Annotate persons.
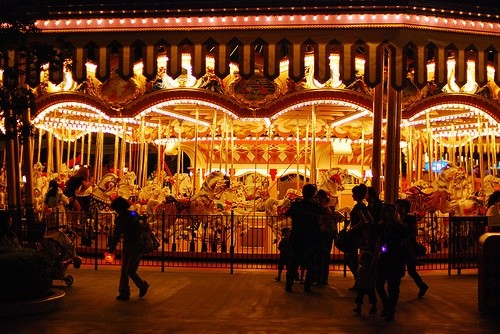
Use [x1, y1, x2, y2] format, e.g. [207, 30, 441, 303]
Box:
[0, 215, 23, 249]
[44, 167, 97, 246]
[105, 196, 160, 300]
[274, 184, 429, 321]
[485, 191, 500, 215]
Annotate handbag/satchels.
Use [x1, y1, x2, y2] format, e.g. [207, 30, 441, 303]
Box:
[335, 229, 360, 252]
[416, 241, 426, 258]
[137, 226, 160, 253]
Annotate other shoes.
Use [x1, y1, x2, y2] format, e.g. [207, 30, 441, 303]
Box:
[115, 294, 129, 301]
[284, 284, 291, 291]
[349, 286, 359, 291]
[379, 302, 395, 321]
[138, 280, 150, 298]
[275, 278, 280, 282]
[369, 306, 377, 315]
[352, 306, 361, 314]
[304, 286, 312, 295]
[418, 283, 429, 298]
[295, 273, 299, 282]
[299, 278, 304, 281]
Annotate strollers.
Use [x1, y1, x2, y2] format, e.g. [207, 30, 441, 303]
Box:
[26, 225, 75, 287]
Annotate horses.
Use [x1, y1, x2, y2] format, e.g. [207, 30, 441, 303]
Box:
[0, 162, 500, 256]
